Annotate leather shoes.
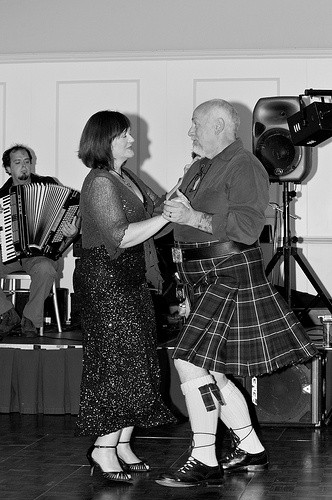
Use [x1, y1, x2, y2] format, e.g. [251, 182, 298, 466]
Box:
[162, 456, 225, 488]
[218, 445, 268, 472]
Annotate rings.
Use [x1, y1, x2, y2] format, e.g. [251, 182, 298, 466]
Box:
[169, 212, 172, 218]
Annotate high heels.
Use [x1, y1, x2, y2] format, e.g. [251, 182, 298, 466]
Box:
[114, 441, 151, 471]
[86, 442, 132, 481]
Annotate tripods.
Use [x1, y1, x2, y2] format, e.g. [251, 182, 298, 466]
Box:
[263, 184, 332, 313]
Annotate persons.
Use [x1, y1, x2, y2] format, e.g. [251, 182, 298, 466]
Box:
[0, 145, 80, 338]
[155, 99, 319, 487]
[73, 110, 190, 481]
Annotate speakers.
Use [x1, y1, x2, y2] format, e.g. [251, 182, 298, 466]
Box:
[246, 360, 313, 427]
[251, 97, 312, 184]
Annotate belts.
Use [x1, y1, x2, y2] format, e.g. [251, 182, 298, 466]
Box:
[171, 238, 264, 262]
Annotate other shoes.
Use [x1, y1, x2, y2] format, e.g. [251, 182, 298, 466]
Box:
[63, 320, 81, 332]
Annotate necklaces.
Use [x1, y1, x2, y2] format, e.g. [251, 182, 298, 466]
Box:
[110, 168, 131, 188]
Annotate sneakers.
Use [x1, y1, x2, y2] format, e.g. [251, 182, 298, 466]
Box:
[20, 315, 37, 338]
[0, 307, 21, 336]
